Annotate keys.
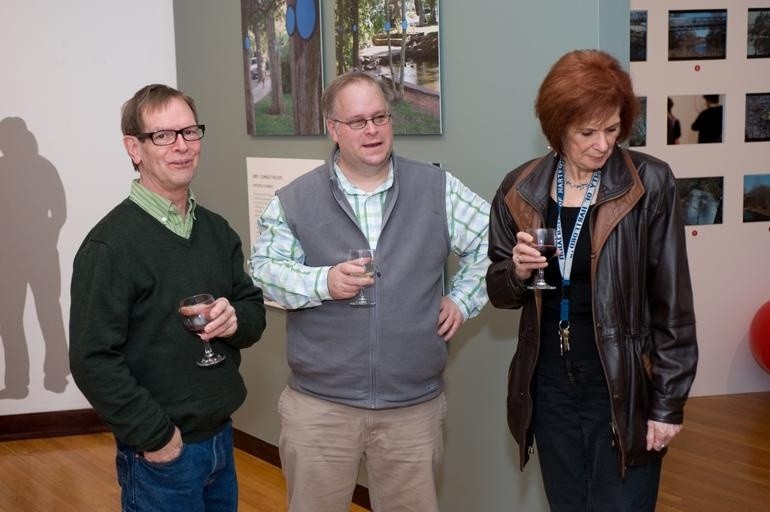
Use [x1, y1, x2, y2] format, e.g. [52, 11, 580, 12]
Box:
[557, 319, 571, 357]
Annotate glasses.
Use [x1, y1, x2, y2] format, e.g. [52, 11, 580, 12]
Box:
[333, 113, 391, 129]
[137, 125, 205, 146]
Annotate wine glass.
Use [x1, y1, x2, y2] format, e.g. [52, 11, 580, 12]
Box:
[522, 226, 562, 289]
[347, 251, 380, 308]
[179, 296, 228, 367]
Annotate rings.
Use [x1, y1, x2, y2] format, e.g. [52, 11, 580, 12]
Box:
[656, 440, 665, 448]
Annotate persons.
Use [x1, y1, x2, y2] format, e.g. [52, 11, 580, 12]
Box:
[69, 86, 267, 512]
[485, 49, 698, 512]
[248, 73, 493, 512]
[665, 94, 723, 145]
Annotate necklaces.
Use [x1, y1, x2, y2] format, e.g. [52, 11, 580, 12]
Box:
[562, 158, 592, 190]
